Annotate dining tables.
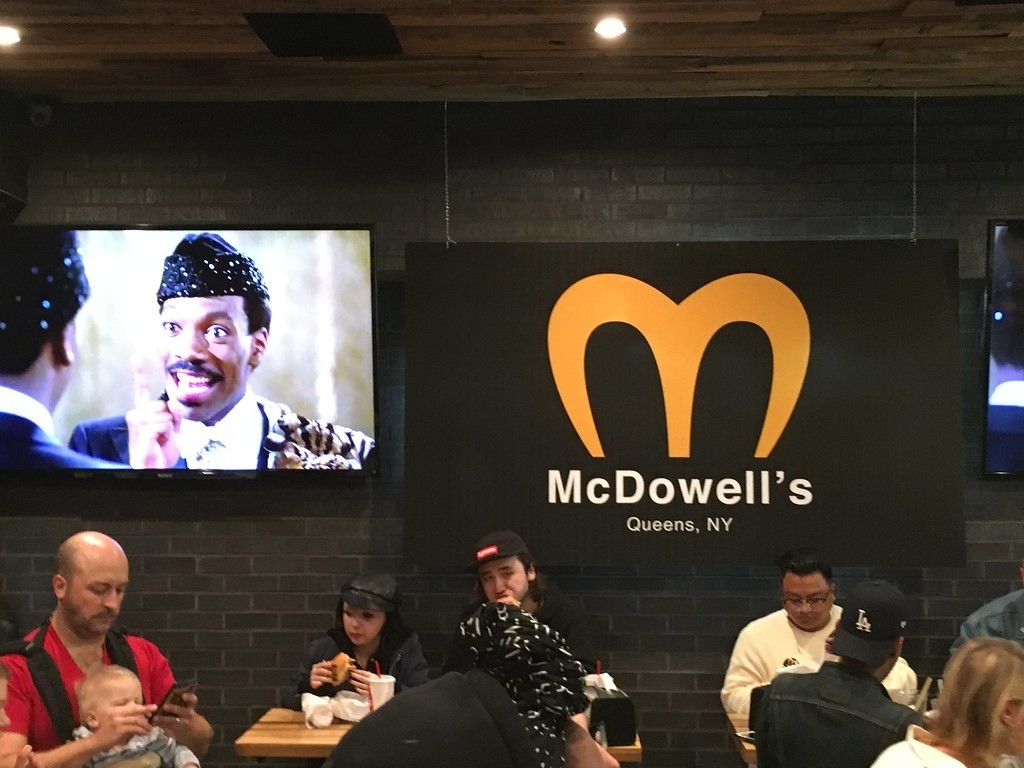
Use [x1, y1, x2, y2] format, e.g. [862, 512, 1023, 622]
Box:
[235, 707, 643, 768]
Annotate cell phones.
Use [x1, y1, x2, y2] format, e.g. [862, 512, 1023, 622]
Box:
[149, 680, 198, 725]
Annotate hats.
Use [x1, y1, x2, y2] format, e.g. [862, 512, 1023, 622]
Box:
[340, 570, 403, 613]
[463, 530, 528, 571]
[157, 231, 268, 305]
[322, 670, 539, 768]
[830, 580, 909, 668]
[0, 231, 90, 349]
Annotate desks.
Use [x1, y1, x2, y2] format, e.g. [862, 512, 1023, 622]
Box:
[726, 710, 758, 764]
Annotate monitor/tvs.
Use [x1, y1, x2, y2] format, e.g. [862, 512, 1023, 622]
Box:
[983, 216, 1024, 480]
[0, 223, 380, 480]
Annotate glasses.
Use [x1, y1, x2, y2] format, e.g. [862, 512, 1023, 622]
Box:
[781, 583, 831, 612]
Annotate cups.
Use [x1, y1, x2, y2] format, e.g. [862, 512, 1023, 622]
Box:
[368, 674, 397, 713]
[897, 686, 940, 716]
[584, 673, 617, 742]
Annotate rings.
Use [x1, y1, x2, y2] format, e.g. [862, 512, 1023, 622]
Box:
[176, 718, 180, 722]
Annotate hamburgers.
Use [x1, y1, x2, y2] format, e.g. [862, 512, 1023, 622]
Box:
[331, 652, 355, 684]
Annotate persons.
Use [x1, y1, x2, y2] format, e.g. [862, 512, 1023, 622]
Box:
[0, 531, 213, 768]
[0, 663, 39, 767]
[720, 548, 918, 712]
[755, 580, 925, 768]
[0, 231, 127, 470]
[65, 665, 200, 768]
[323, 602, 619, 768]
[436, 531, 602, 676]
[949, 556, 1024, 652]
[869, 637, 1024, 768]
[291, 572, 433, 710]
[68, 234, 374, 470]
